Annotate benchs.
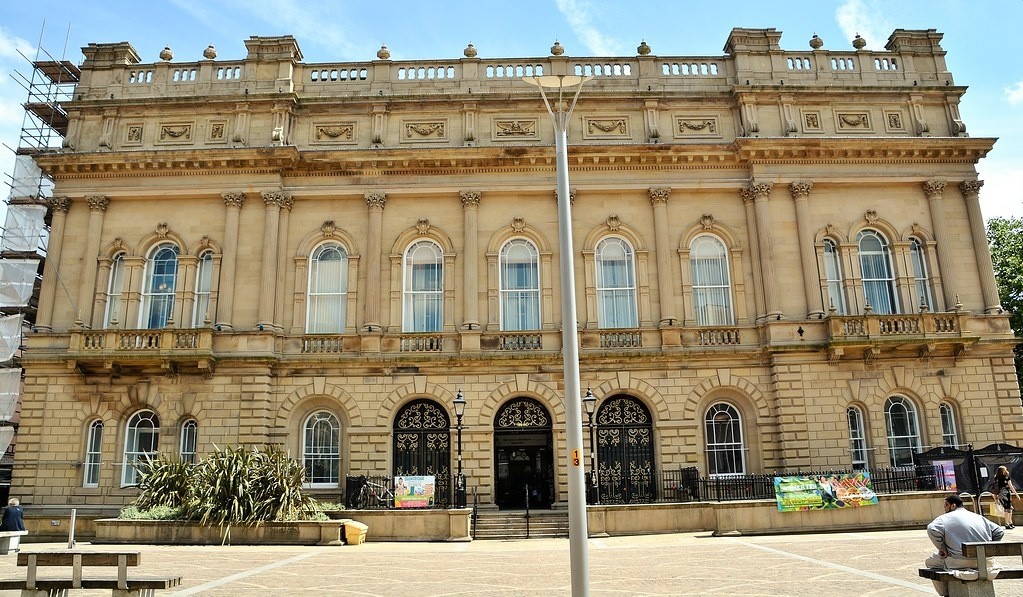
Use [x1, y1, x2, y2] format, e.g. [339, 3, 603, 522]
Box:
[0, 530, 28, 555]
[919, 542, 1023, 597]
[0, 551, 183, 597]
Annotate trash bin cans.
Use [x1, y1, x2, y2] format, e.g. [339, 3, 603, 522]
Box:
[344, 521, 369, 545]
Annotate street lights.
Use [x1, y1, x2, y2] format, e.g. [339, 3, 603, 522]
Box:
[452, 388, 467, 509]
[582, 386, 599, 505]
[522, 75, 595, 597]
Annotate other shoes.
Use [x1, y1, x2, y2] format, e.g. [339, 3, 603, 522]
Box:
[1005, 523, 1015, 529]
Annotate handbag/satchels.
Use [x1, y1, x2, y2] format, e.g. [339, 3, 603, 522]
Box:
[991, 481, 999, 495]
[989, 502, 1005, 518]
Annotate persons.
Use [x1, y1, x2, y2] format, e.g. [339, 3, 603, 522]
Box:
[992, 465, 1021, 529]
[0, 498, 25, 552]
[925, 494, 1005, 597]
[818, 476, 836, 503]
[395, 477, 407, 496]
